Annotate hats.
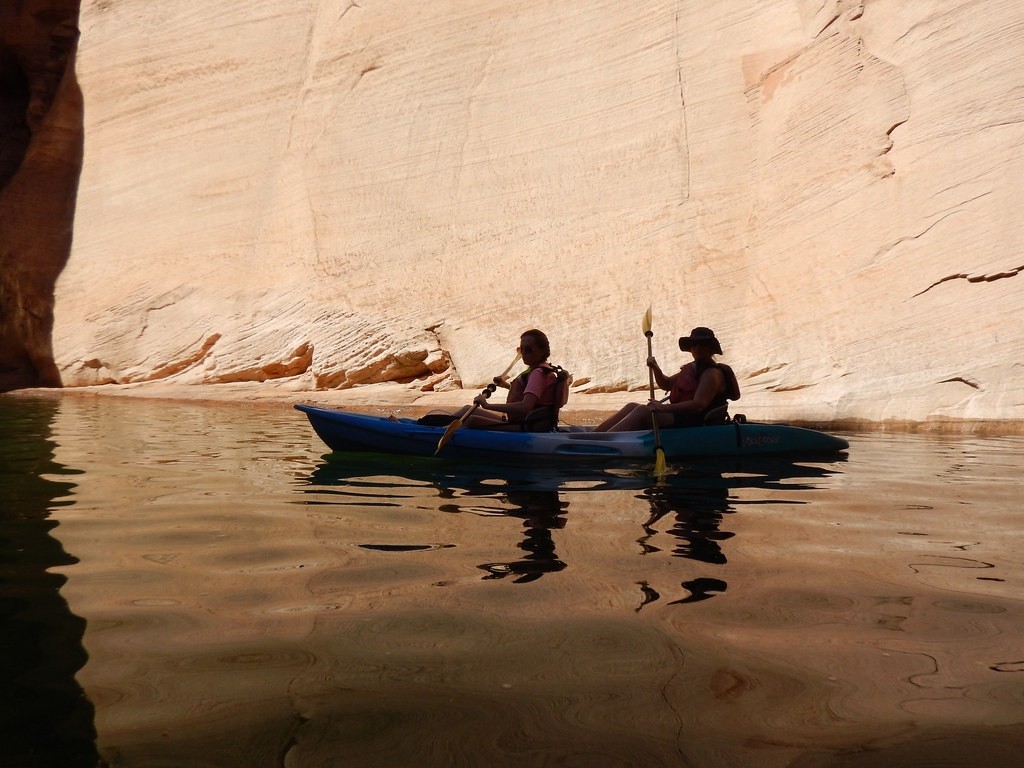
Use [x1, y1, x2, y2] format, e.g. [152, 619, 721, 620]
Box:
[679, 327, 722, 355]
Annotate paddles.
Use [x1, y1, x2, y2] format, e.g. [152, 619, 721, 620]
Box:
[641, 301, 666, 479]
[434, 350, 525, 455]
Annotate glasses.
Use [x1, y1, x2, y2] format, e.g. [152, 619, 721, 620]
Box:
[691, 342, 709, 348]
[517, 346, 542, 354]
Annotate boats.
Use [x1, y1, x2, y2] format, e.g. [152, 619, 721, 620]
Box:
[293, 404, 852, 468]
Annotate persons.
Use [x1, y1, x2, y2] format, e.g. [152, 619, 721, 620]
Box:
[388, 329, 571, 424]
[597, 327, 741, 431]
